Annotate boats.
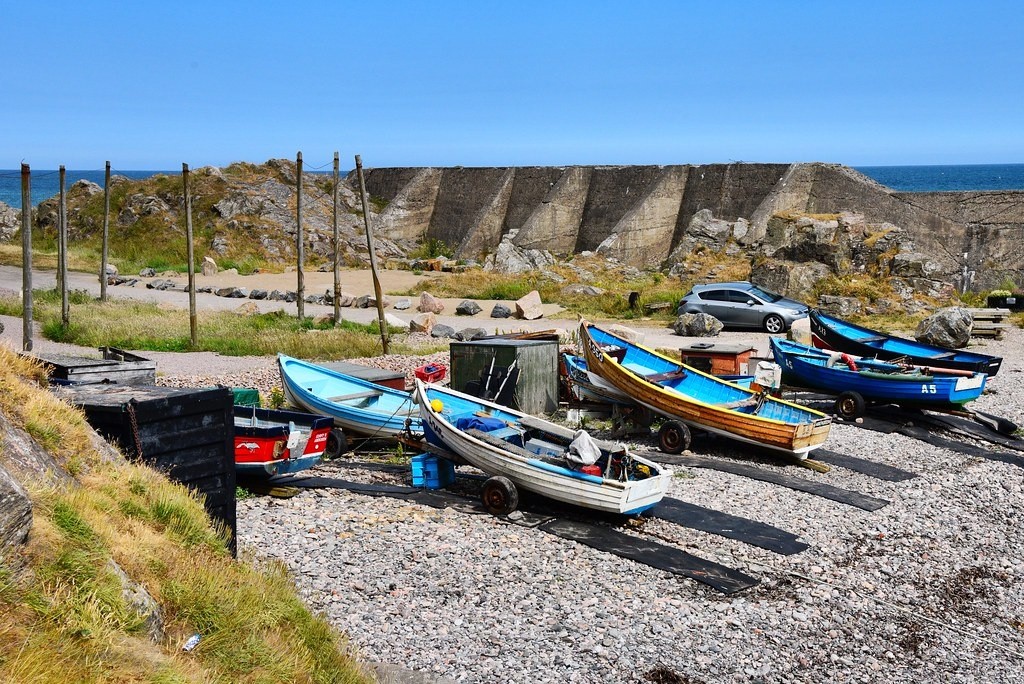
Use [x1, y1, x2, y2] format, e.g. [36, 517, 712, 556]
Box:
[275, 350, 428, 440]
[806, 305, 1004, 381]
[561, 352, 757, 407]
[578, 316, 833, 455]
[413, 376, 672, 517]
[232, 402, 334, 476]
[768, 333, 990, 406]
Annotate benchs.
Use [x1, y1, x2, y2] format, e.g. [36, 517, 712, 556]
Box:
[973, 316, 1008, 323]
[969, 324, 1011, 340]
[327, 390, 384, 403]
[714, 397, 760, 410]
[488, 424, 532, 438]
[932, 351, 956, 360]
[600, 344, 627, 352]
[855, 335, 887, 342]
[647, 370, 688, 383]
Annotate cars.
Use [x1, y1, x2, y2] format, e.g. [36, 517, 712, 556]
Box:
[678, 281, 811, 334]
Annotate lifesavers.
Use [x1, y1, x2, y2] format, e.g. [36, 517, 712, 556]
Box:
[826, 352, 858, 371]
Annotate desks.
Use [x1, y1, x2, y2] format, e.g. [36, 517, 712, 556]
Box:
[962, 308, 1011, 323]
[206, 386, 263, 408]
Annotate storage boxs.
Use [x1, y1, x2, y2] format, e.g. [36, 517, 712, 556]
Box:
[415, 362, 446, 383]
